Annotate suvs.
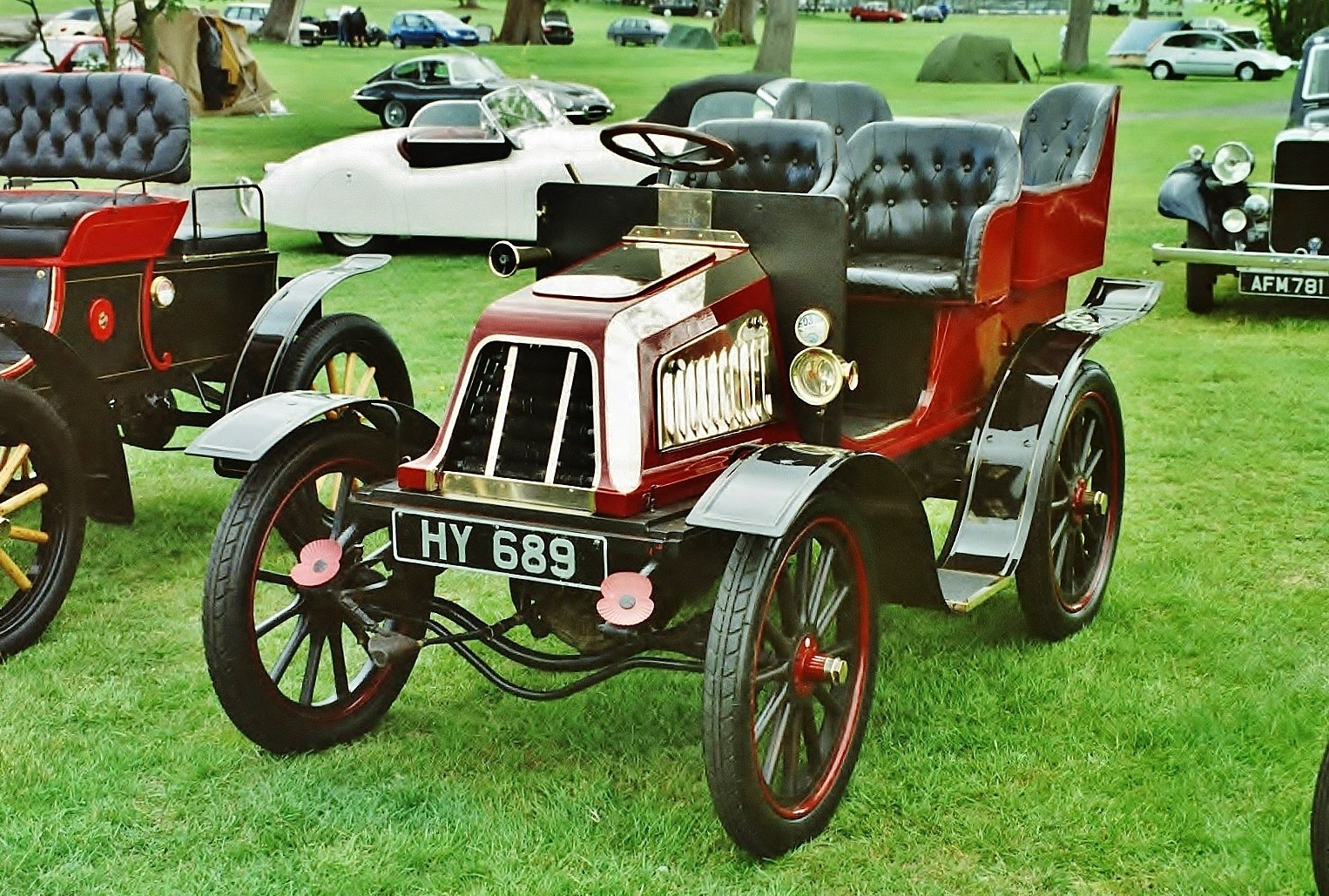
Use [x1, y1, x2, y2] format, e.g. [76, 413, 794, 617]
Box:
[222, 1, 321, 47]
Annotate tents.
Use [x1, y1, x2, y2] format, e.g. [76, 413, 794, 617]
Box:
[88, 0, 280, 122]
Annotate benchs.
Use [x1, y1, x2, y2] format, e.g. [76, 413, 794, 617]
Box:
[0, 71, 192, 258]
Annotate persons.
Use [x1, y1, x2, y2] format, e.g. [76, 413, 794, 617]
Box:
[338, 11, 350, 47]
[198, 17, 224, 111]
[350, 6, 367, 48]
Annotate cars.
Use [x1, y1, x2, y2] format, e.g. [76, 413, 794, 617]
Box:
[850, 2, 908, 23]
[912, 5, 944, 23]
[181, 80, 1165, 859]
[0, 7, 179, 98]
[387, 9, 480, 50]
[540, 9, 574, 45]
[0, 71, 417, 664]
[607, 16, 670, 47]
[299, 5, 384, 47]
[621, 0, 726, 18]
[1150, 23, 1329, 317]
[1143, 30, 1294, 82]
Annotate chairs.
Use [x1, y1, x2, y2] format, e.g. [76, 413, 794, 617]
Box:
[670, 78, 1123, 302]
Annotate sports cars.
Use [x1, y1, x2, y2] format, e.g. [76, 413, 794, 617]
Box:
[350, 54, 614, 136]
